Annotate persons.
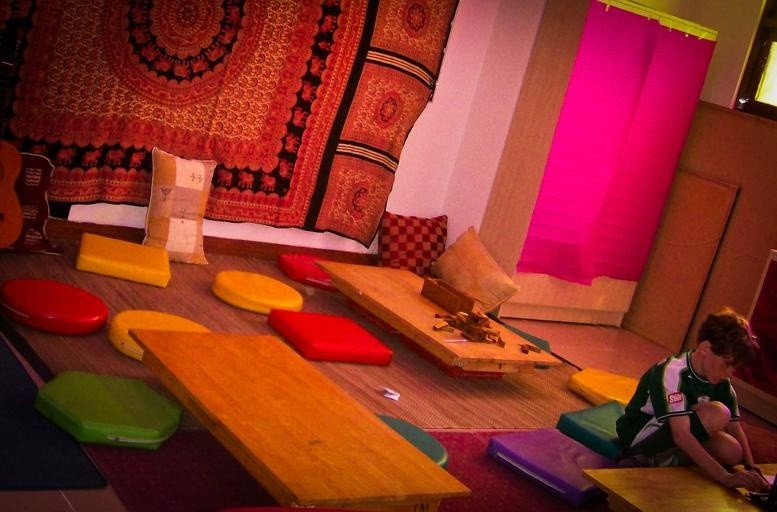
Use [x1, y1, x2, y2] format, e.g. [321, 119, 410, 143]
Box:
[616, 304, 775, 501]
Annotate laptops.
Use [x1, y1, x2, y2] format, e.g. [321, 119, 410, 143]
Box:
[748, 473, 777, 512]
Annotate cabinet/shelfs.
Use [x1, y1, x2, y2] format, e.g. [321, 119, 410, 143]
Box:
[473, 0, 720, 328]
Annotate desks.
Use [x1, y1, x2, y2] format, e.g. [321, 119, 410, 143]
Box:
[583, 461, 776, 512]
[317, 258, 561, 387]
[127, 318, 471, 509]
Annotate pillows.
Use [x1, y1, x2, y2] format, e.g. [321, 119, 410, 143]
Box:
[378, 209, 521, 317]
[141, 147, 217, 265]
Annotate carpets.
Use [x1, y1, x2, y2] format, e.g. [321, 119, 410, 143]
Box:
[91, 429, 608, 511]
[0, 329, 108, 491]
